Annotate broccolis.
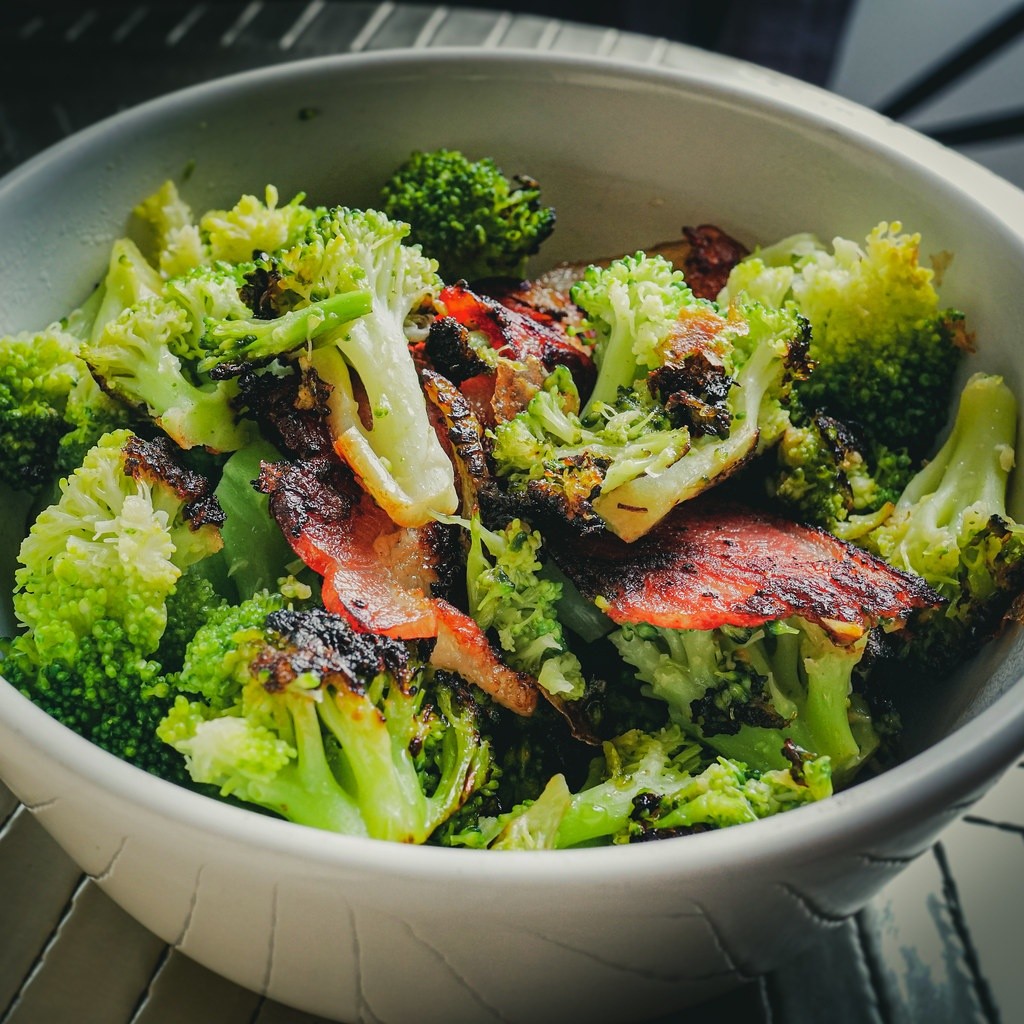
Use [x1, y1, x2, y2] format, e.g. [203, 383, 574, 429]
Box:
[1, 147, 1024, 845]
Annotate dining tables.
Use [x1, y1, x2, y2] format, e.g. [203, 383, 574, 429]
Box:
[0, 4, 1024, 1023]
